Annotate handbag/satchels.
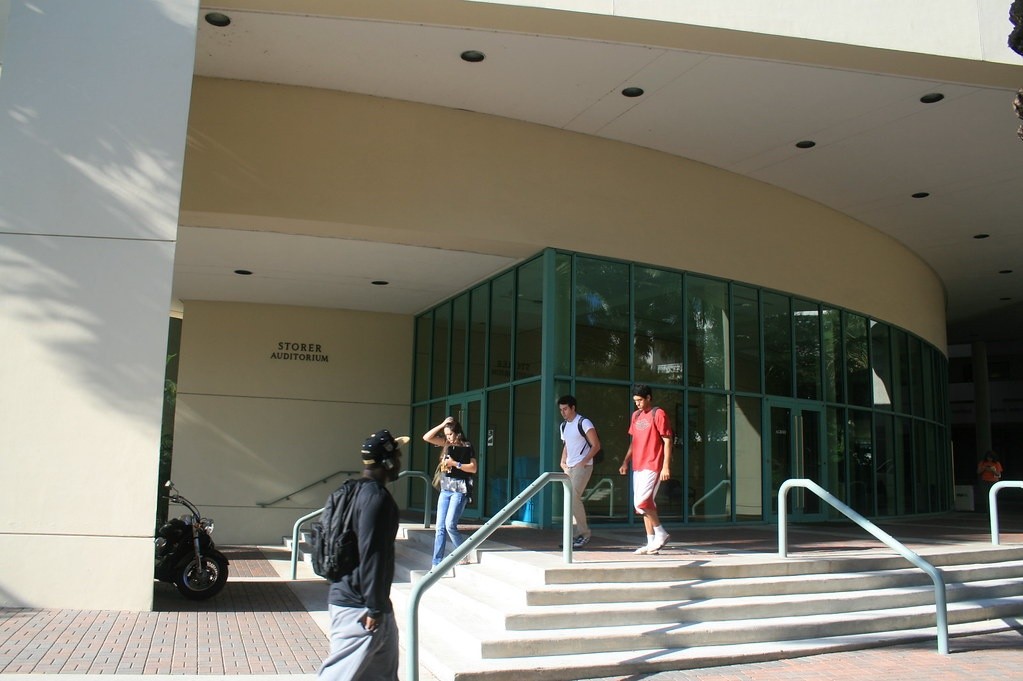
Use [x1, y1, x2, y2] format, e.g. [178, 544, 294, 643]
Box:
[432, 469, 441, 491]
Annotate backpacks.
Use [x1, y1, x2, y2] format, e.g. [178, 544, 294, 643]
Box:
[311, 477, 370, 582]
[562, 417, 604, 464]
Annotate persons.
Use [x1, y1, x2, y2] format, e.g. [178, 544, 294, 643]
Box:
[318, 430, 410, 681]
[619, 385, 672, 555]
[423, 417, 477, 576]
[977, 452, 1003, 517]
[558, 394, 601, 548]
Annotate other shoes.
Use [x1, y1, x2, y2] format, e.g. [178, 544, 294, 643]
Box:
[456, 556, 468, 564]
[421, 570, 433, 578]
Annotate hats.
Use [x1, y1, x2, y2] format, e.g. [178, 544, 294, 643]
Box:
[361, 429, 411, 464]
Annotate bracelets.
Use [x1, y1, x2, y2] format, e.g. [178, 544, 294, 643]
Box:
[457, 462, 461, 469]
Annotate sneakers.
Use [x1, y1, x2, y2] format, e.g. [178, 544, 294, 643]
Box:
[573, 535, 592, 548]
[559, 537, 578, 549]
[634, 544, 658, 555]
[646, 531, 670, 552]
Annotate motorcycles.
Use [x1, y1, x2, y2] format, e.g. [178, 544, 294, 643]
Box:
[155, 480, 230, 602]
